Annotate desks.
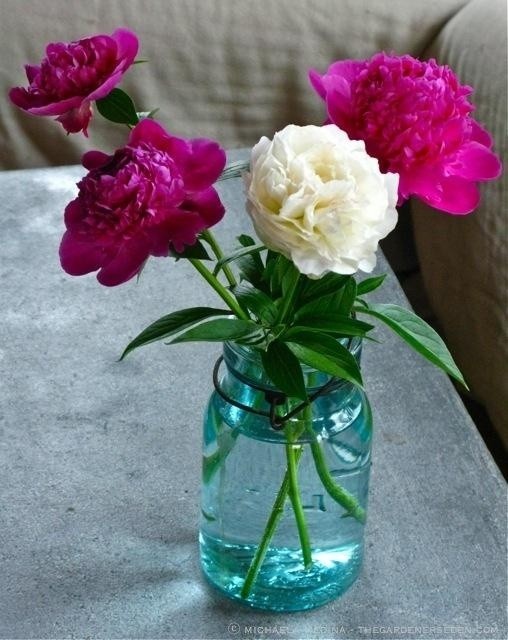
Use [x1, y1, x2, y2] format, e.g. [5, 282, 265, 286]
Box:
[1, 145, 508, 638]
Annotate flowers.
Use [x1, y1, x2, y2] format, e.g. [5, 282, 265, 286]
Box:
[9, 25, 504, 596]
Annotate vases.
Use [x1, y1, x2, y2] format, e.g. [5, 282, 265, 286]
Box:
[194, 332, 373, 613]
